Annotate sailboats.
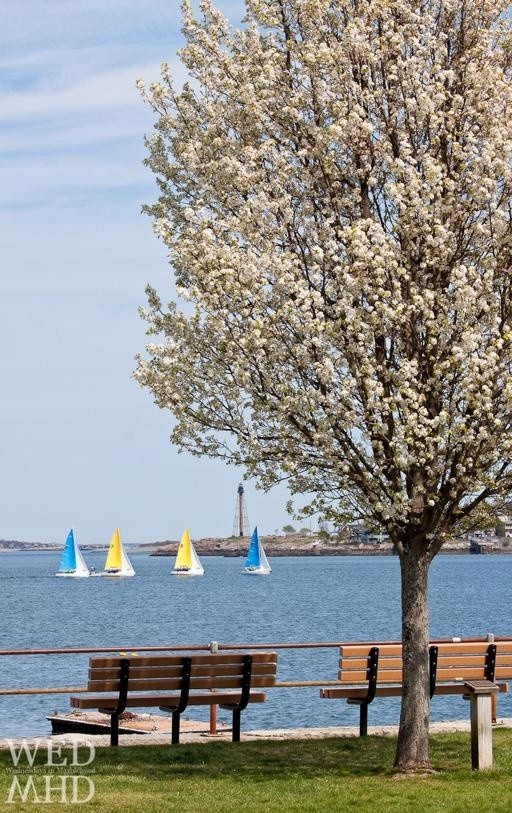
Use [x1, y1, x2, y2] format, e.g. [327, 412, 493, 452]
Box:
[93, 525, 136, 576]
[54, 529, 90, 578]
[171, 528, 204, 576]
[241, 526, 273, 575]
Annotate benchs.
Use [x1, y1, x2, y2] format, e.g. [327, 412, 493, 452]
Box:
[319, 640, 512, 738]
[68, 650, 279, 748]
